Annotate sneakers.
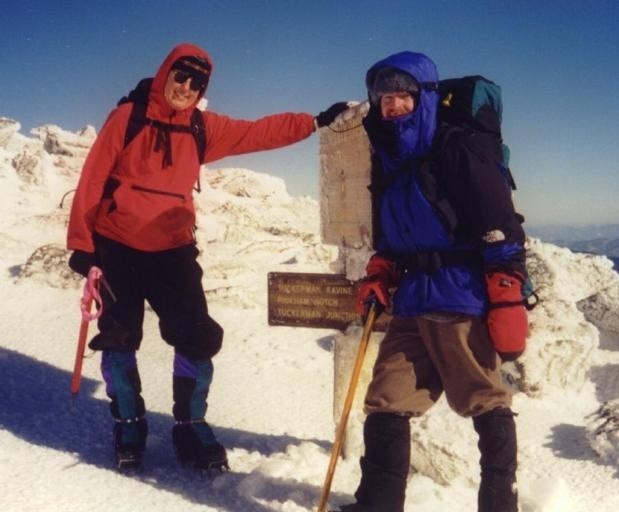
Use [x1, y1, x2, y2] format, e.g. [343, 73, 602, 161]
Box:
[177, 454, 227, 473]
[116, 439, 142, 470]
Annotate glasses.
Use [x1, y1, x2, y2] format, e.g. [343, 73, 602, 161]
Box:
[173, 69, 202, 91]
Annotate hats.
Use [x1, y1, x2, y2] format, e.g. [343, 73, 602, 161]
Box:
[171, 55, 210, 84]
[374, 68, 418, 96]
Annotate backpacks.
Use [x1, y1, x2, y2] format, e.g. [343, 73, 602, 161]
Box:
[423, 75, 525, 224]
[117, 77, 205, 167]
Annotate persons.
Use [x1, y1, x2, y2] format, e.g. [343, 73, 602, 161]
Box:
[66, 43, 361, 468]
[339, 49, 541, 512]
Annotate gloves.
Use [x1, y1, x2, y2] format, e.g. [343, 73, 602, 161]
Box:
[486, 268, 528, 360]
[356, 256, 396, 325]
[69, 249, 99, 277]
[317, 101, 349, 127]
